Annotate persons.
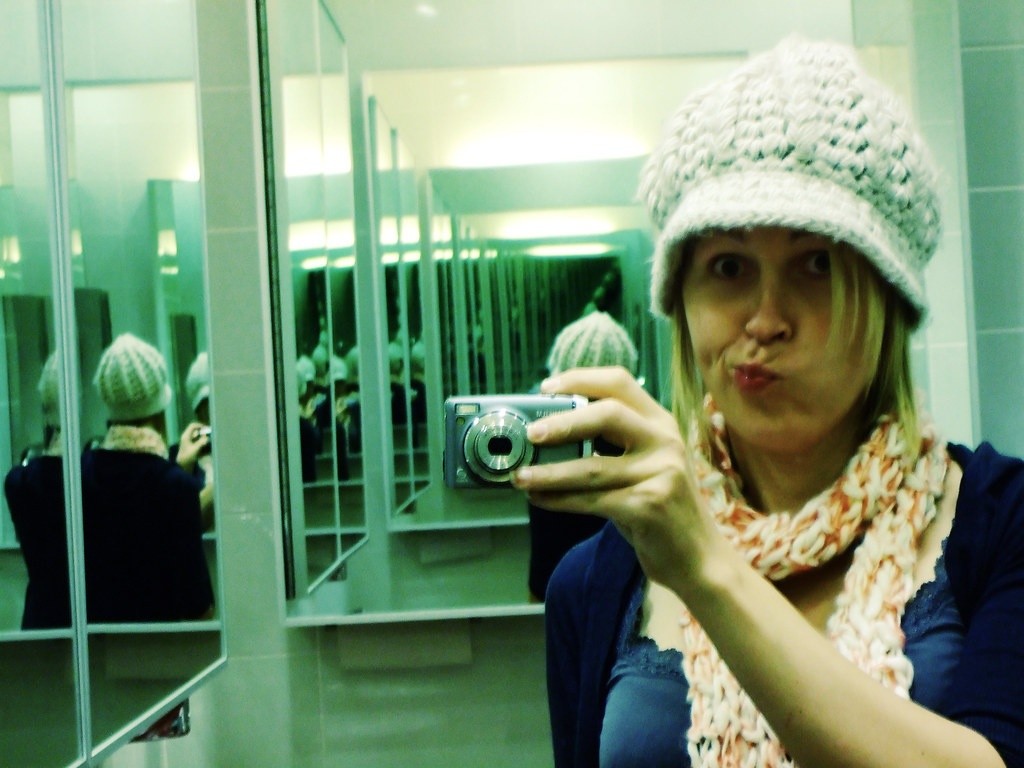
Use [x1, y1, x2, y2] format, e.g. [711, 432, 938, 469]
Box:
[524, 310, 641, 603]
[4, 330, 426, 639]
[508, 34, 1024, 768]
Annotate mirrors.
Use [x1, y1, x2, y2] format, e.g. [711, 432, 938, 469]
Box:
[0, 1, 229, 768]
[1, 0, 650, 643]
[254, 1, 979, 630]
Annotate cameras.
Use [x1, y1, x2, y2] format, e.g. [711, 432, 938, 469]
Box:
[342, 393, 359, 406]
[312, 394, 327, 409]
[191, 427, 213, 454]
[442, 393, 591, 490]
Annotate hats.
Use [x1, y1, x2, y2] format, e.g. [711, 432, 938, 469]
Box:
[546, 312, 638, 381]
[298, 334, 428, 381]
[638, 36, 941, 327]
[39, 350, 61, 424]
[95, 333, 172, 420]
[186, 352, 210, 410]
